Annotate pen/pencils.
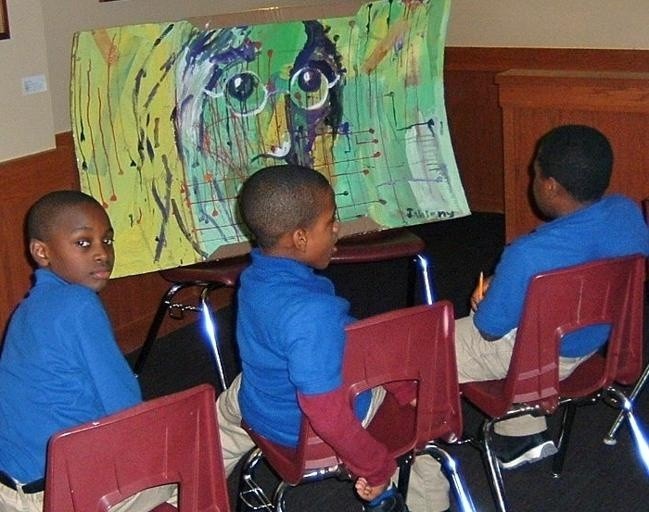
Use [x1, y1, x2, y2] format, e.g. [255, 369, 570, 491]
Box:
[478, 270, 484, 300]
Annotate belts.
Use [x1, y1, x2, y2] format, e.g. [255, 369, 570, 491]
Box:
[0, 470, 45, 494]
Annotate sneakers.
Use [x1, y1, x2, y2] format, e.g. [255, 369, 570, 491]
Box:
[362, 480, 407, 512]
[495, 428, 559, 471]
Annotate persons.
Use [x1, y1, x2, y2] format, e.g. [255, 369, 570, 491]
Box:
[454, 120, 649, 474]
[211, 161, 456, 511]
[0, 189, 146, 512]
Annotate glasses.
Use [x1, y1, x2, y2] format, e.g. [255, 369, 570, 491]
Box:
[202, 65, 341, 119]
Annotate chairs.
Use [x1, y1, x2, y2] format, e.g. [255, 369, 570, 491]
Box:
[239, 301, 478, 512]
[130, 260, 239, 394]
[465, 252, 649, 512]
[44, 385, 232, 511]
[327, 228, 435, 304]
